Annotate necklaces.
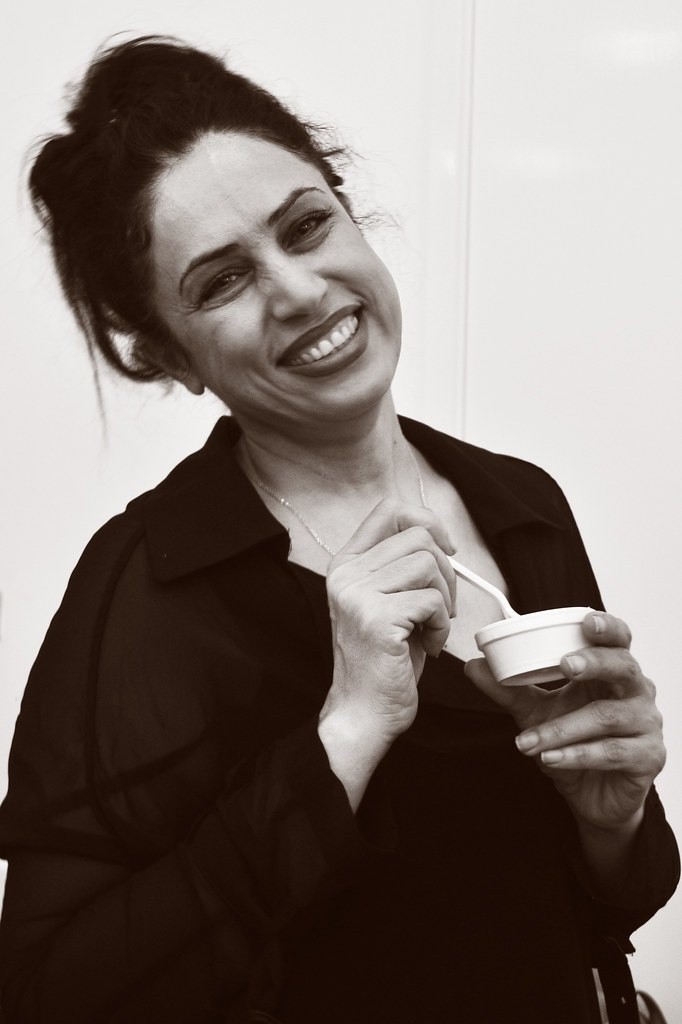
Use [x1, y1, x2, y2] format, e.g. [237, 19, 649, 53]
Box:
[243, 446, 427, 552]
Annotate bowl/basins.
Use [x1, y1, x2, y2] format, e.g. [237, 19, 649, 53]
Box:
[474, 607, 602, 686]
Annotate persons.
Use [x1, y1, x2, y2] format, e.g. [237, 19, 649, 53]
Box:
[0, 33, 681, 1024]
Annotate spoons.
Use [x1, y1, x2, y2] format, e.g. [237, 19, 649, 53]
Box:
[445, 553, 522, 619]
